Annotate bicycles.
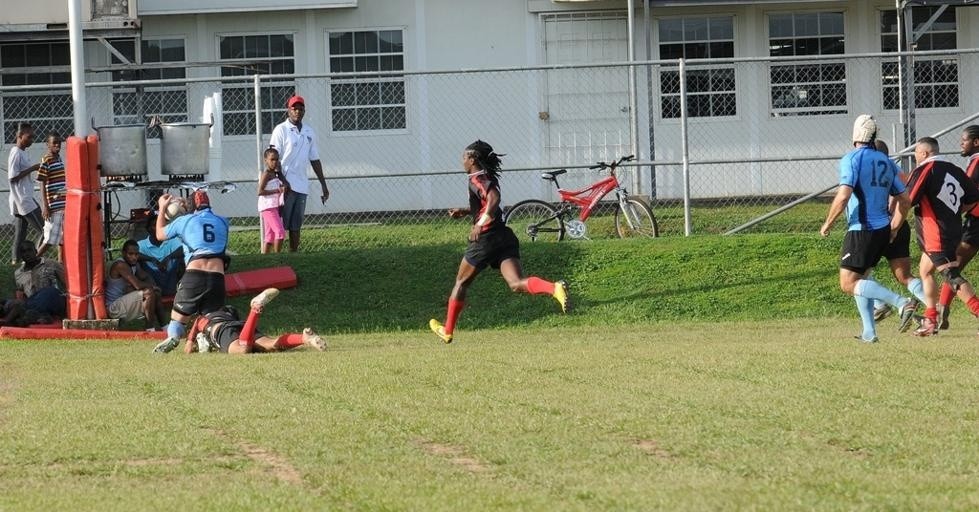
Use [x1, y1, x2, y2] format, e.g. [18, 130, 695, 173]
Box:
[506, 155, 658, 239]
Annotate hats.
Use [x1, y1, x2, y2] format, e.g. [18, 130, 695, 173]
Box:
[288, 96, 304, 107]
[187, 190, 209, 209]
[852, 114, 877, 145]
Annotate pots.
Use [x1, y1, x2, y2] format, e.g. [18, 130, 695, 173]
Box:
[90, 115, 156, 177]
[156, 112, 215, 176]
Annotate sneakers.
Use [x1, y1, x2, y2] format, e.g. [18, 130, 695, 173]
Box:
[153, 337, 178, 354]
[855, 301, 949, 342]
[429, 319, 453, 343]
[303, 328, 326, 350]
[196, 334, 212, 353]
[554, 281, 571, 314]
[250, 288, 279, 307]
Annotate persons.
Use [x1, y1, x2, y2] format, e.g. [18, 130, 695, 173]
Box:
[0, 239, 65, 326]
[819, 114, 920, 344]
[912, 125, 979, 330]
[903, 137, 979, 339]
[104, 189, 327, 355]
[35, 132, 67, 266]
[866, 139, 950, 329]
[429, 140, 571, 344]
[256, 145, 291, 255]
[7, 122, 45, 266]
[269, 96, 329, 254]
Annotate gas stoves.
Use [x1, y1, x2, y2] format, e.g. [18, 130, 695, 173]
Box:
[166, 174, 206, 184]
[104, 174, 143, 184]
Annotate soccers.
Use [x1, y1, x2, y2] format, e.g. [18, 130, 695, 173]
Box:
[195, 332, 211, 352]
[165, 199, 188, 219]
